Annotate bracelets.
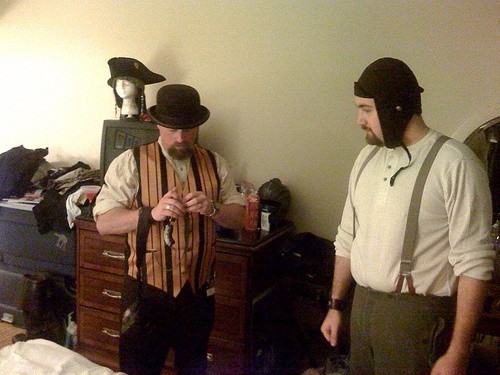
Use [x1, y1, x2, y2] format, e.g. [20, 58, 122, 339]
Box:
[327, 299, 344, 309]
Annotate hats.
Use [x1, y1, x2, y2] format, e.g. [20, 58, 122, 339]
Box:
[106, 56, 167, 85]
[354, 57, 423, 149]
[147, 84, 211, 129]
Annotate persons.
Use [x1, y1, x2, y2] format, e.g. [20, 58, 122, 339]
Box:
[93, 84, 247, 375]
[107, 57, 167, 115]
[320, 58, 496, 375]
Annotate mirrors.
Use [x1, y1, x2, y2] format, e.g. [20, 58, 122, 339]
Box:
[450, 108, 500, 225]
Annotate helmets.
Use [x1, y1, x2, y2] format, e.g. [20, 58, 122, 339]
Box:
[258, 178, 291, 230]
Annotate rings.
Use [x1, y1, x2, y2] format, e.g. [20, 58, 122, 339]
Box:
[167, 205, 170, 210]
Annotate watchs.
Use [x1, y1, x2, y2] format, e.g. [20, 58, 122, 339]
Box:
[208, 200, 219, 217]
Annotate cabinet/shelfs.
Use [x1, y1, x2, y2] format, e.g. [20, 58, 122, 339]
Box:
[76, 216, 296, 375]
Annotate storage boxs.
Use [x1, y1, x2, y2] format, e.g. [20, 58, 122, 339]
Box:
[0, 195, 77, 276]
